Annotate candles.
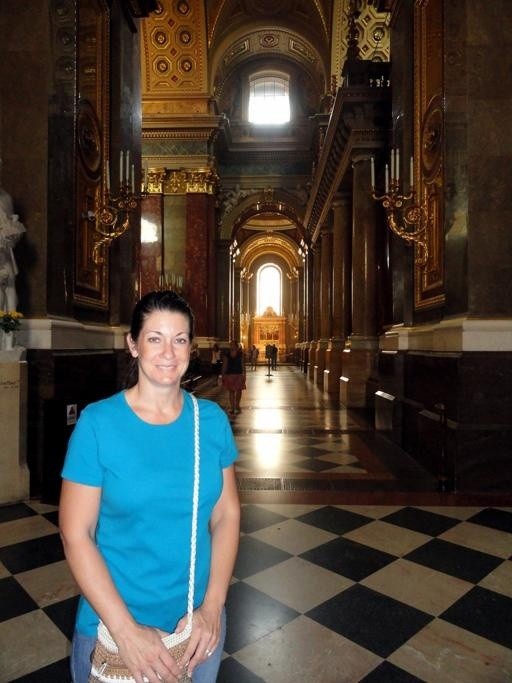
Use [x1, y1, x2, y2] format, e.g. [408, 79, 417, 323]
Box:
[370, 148, 415, 193]
[103, 149, 149, 195]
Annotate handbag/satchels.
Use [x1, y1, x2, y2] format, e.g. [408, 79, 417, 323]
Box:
[87, 616, 193, 683]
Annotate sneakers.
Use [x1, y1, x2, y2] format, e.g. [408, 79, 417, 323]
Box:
[227, 405, 241, 414]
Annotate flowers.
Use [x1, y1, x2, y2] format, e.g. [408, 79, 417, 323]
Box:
[1, 311, 22, 333]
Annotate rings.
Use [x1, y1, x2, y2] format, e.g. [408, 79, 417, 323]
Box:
[207, 651, 214, 657]
[205, 648, 210, 653]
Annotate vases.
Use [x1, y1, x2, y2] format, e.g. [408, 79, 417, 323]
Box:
[5, 334, 14, 350]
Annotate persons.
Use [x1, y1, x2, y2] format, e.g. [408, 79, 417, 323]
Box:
[249, 344, 259, 371]
[208, 343, 222, 386]
[221, 340, 247, 413]
[61, 290, 242, 683]
[270, 343, 278, 370]
